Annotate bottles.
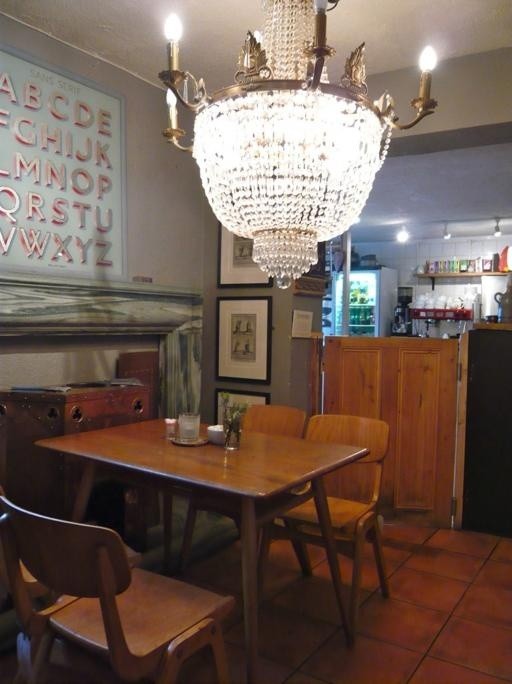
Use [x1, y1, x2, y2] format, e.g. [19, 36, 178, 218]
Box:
[349, 307, 370, 324]
[495, 286, 512, 323]
[350, 280, 369, 304]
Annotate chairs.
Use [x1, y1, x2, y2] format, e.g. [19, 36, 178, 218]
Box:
[177, 404, 389, 648]
[0, 495, 235, 683]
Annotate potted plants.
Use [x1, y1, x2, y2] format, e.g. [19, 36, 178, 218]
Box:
[218, 392, 249, 452]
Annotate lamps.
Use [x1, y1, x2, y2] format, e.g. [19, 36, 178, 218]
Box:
[159, 0, 438, 291]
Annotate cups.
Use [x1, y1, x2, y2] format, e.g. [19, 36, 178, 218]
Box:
[179, 412, 201, 442]
[414, 318, 473, 339]
[416, 292, 456, 310]
[485, 316, 498, 324]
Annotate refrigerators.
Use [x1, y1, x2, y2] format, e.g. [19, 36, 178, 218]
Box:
[334, 268, 398, 337]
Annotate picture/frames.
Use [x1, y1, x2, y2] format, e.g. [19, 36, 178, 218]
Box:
[0, 42, 128, 283]
[214, 222, 273, 431]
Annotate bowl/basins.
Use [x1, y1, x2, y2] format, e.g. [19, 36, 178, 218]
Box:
[207, 425, 241, 445]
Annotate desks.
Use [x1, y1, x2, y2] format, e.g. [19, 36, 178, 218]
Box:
[33, 418, 371, 683]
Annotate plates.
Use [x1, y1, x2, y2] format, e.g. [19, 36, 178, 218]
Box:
[171, 438, 209, 447]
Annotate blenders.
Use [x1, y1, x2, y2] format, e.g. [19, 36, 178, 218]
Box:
[391, 286, 413, 335]
